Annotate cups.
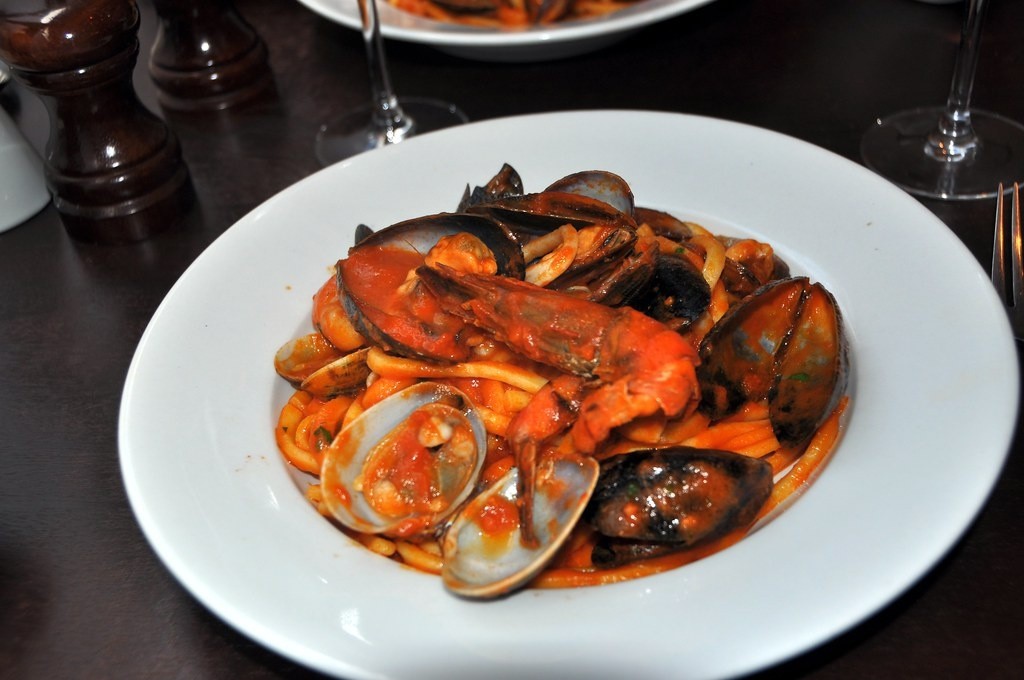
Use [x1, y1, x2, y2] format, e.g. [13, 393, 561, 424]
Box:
[1, 106, 50, 236]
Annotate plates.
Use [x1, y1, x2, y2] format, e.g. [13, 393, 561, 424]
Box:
[115, 111, 1023, 679]
[299, 0, 710, 45]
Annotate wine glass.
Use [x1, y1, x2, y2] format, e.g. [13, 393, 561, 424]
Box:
[859, 0, 1024, 199]
[313, 0, 470, 161]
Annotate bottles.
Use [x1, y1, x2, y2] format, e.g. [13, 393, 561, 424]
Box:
[147, 0, 270, 112]
[0, 0, 186, 226]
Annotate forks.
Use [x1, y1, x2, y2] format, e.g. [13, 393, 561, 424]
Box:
[992, 183, 1024, 380]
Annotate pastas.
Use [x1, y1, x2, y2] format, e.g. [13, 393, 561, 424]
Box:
[276, 225, 850, 585]
[386, 0, 636, 26]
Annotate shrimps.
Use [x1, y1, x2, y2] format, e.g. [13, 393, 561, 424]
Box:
[427, 261, 698, 456]
